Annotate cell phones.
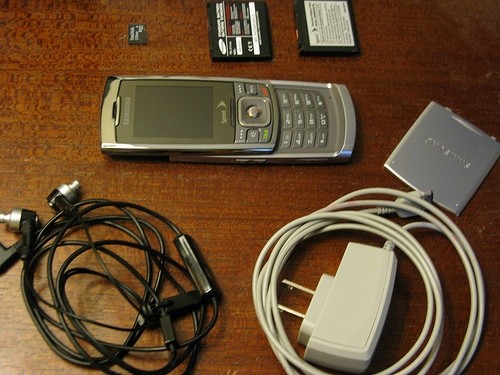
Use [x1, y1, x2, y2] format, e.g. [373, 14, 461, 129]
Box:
[100, 74, 358, 164]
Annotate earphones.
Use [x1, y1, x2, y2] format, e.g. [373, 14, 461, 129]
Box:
[45, 180, 81, 213]
[0, 209, 39, 257]
